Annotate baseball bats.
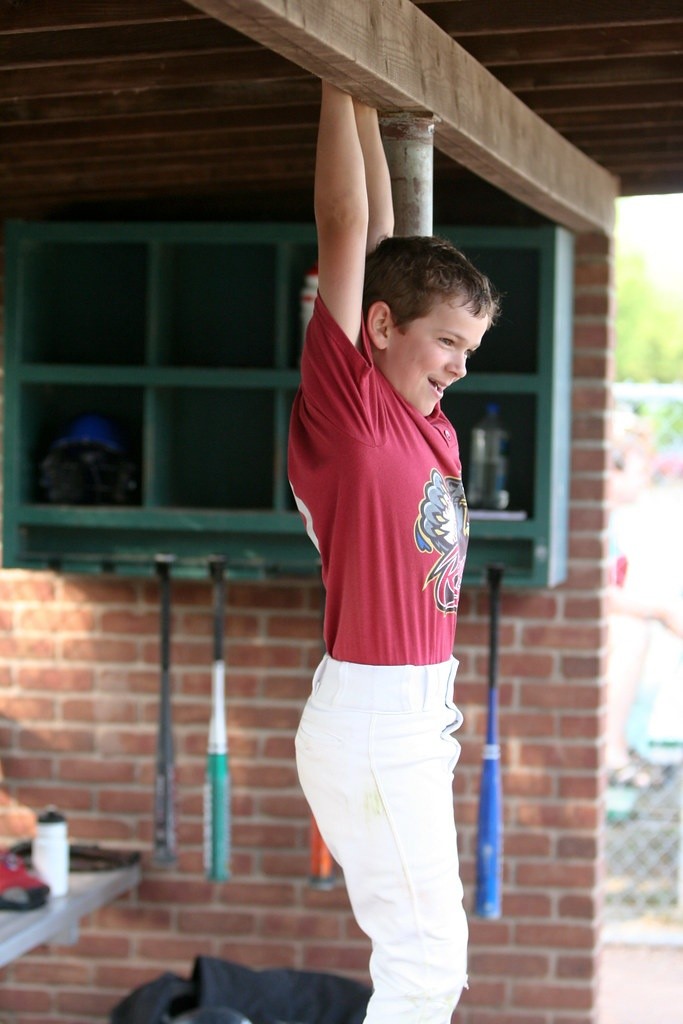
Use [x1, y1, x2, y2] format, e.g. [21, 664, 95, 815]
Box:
[304, 817, 337, 893]
[471, 562, 507, 920]
[142, 560, 177, 865]
[199, 558, 232, 880]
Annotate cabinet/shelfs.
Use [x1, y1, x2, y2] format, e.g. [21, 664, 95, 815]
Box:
[0, 221, 573, 594]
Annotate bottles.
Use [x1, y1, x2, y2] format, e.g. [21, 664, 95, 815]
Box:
[464, 406, 511, 511]
[28, 803, 70, 900]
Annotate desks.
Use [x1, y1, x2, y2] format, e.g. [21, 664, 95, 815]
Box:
[0, 865, 145, 972]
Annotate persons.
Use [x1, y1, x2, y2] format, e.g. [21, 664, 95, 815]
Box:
[287, 78, 496, 1024]
